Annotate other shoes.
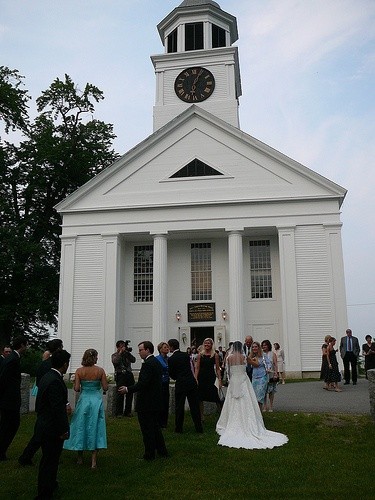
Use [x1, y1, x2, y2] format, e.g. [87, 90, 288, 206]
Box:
[113, 414, 122, 419]
[262, 407, 267, 412]
[282, 380, 285, 384]
[136, 457, 148, 462]
[268, 408, 272, 412]
[353, 382, 357, 385]
[124, 413, 134, 417]
[20, 460, 35, 466]
[344, 382, 350, 385]
[323, 384, 342, 392]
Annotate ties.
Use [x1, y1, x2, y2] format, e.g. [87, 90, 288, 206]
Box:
[348, 337, 351, 351]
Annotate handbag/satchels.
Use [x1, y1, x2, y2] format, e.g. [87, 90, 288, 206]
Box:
[266, 371, 280, 383]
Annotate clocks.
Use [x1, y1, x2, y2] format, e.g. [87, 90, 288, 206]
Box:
[173, 67, 215, 103]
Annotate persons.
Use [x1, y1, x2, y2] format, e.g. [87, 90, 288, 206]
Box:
[112, 340, 136, 418]
[215, 341, 289, 450]
[63, 348, 109, 471]
[362, 335, 375, 379]
[36, 349, 71, 500]
[195, 338, 222, 405]
[155, 342, 170, 414]
[319, 335, 342, 392]
[243, 336, 286, 413]
[339, 329, 360, 385]
[0, 336, 63, 465]
[187, 342, 234, 387]
[167, 339, 203, 434]
[118, 341, 166, 462]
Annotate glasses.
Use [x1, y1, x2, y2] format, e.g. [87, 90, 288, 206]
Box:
[139, 349, 144, 352]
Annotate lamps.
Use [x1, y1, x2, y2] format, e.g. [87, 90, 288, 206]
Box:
[176, 311, 181, 321]
[221, 310, 227, 320]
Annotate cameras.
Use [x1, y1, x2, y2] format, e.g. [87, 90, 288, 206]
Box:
[123, 341, 132, 352]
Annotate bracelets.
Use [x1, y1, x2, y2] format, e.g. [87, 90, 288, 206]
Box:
[218, 379, 221, 381]
